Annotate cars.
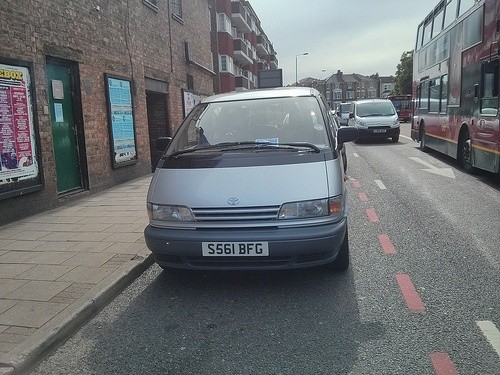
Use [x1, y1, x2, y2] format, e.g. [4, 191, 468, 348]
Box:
[348, 98, 401, 144]
[336, 102, 352, 126]
[469, 108, 499, 132]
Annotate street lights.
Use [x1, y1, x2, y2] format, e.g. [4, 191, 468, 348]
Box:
[295, 52, 309, 87]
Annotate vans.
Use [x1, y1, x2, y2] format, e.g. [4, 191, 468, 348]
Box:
[143, 87, 360, 273]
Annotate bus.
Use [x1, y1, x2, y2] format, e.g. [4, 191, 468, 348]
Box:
[388, 94, 411, 123]
[411, 0, 500, 174]
